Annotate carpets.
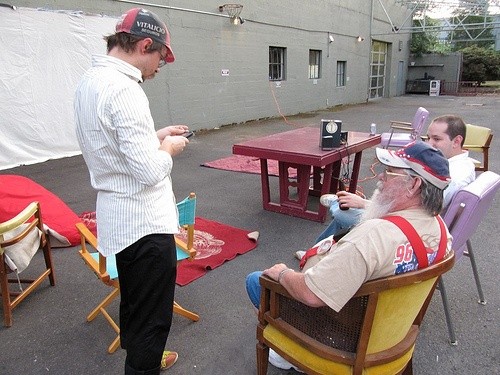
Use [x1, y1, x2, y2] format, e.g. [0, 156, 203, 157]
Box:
[200, 153, 352, 178]
[175, 216, 259, 287]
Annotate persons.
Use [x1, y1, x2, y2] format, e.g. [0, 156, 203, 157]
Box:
[72, 6, 190, 375]
[246, 141, 455, 374]
[295, 114, 475, 260]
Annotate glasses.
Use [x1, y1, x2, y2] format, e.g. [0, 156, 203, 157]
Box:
[158, 50, 167, 67]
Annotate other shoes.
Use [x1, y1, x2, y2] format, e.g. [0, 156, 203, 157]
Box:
[161, 351, 177, 370]
[320, 194, 338, 206]
[268, 348, 305, 373]
[295, 250, 305, 260]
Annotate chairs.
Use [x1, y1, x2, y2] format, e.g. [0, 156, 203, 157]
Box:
[434, 170, 500, 345]
[421, 123, 493, 172]
[74, 191, 197, 354]
[0, 201, 55, 327]
[256, 248, 455, 375]
[381, 106, 430, 150]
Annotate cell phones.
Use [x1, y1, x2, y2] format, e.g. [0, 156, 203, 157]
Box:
[178, 131, 194, 139]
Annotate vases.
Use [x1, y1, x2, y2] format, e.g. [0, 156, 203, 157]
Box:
[411, 62, 416, 66]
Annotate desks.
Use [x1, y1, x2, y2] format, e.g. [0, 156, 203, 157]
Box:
[232, 127, 381, 223]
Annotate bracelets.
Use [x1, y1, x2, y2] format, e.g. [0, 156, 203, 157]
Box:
[277, 268, 295, 282]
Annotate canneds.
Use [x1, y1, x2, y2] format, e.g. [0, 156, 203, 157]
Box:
[370, 123, 376, 135]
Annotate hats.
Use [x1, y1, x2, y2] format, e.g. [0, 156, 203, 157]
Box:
[375, 139, 452, 190]
[115, 7, 176, 63]
